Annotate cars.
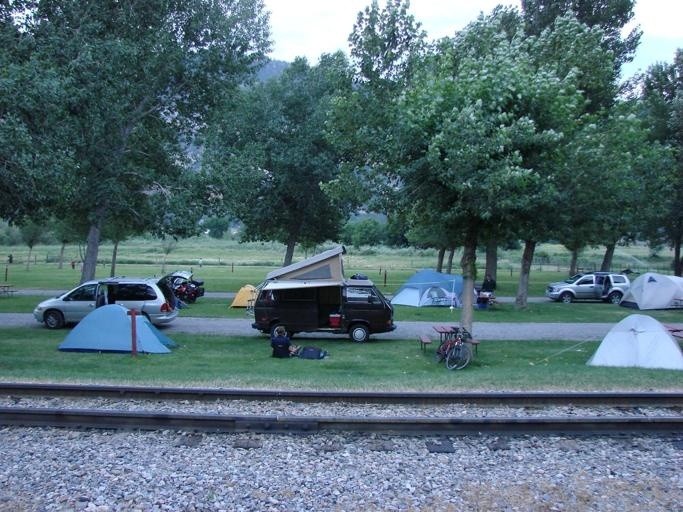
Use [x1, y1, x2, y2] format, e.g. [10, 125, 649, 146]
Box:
[545, 271, 630, 304]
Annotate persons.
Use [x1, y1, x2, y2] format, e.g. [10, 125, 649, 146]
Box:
[482, 274, 497, 292]
[271, 327, 291, 358]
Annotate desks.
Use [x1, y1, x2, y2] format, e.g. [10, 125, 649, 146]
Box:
[0, 284, 16, 295]
[433, 326, 456, 343]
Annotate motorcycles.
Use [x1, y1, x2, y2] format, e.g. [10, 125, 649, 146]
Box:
[169, 276, 205, 303]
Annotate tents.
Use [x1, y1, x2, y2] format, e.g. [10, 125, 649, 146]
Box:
[389, 269, 478, 309]
[57, 303, 179, 354]
[227, 285, 260, 310]
[585, 314, 683, 370]
[619, 273, 683, 311]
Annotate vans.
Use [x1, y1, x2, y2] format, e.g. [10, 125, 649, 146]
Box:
[251, 280, 396, 342]
[33, 270, 194, 329]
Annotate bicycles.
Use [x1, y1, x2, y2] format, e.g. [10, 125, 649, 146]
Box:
[436, 326, 472, 370]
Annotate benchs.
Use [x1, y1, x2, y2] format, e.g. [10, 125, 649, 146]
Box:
[0, 290, 18, 297]
[466, 339, 480, 354]
[419, 335, 431, 353]
